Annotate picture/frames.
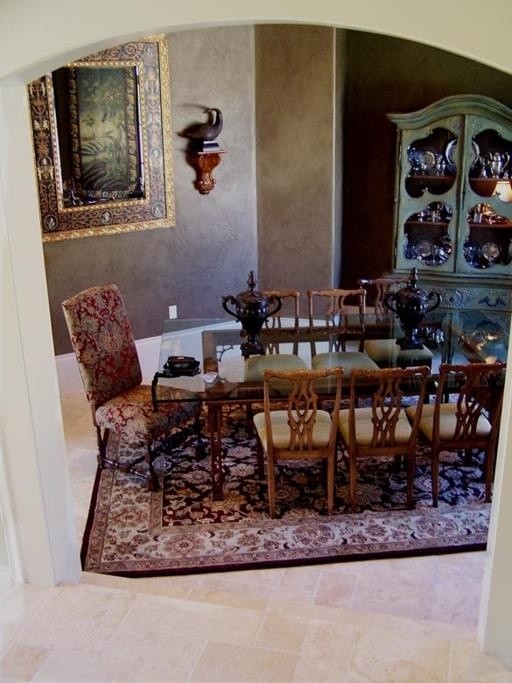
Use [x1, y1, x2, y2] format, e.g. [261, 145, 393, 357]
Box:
[18, 35, 177, 244]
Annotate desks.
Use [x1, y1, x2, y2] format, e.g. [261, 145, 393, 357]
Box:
[153, 310, 509, 499]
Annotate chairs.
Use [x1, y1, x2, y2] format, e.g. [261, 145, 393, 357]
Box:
[359, 274, 435, 406]
[404, 361, 505, 506]
[64, 281, 205, 492]
[243, 289, 309, 432]
[329, 367, 431, 511]
[304, 287, 382, 411]
[251, 365, 343, 519]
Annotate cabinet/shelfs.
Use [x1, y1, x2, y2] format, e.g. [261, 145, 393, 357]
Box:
[385, 93, 511, 371]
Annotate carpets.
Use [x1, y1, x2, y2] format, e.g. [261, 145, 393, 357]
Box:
[79, 391, 493, 580]
